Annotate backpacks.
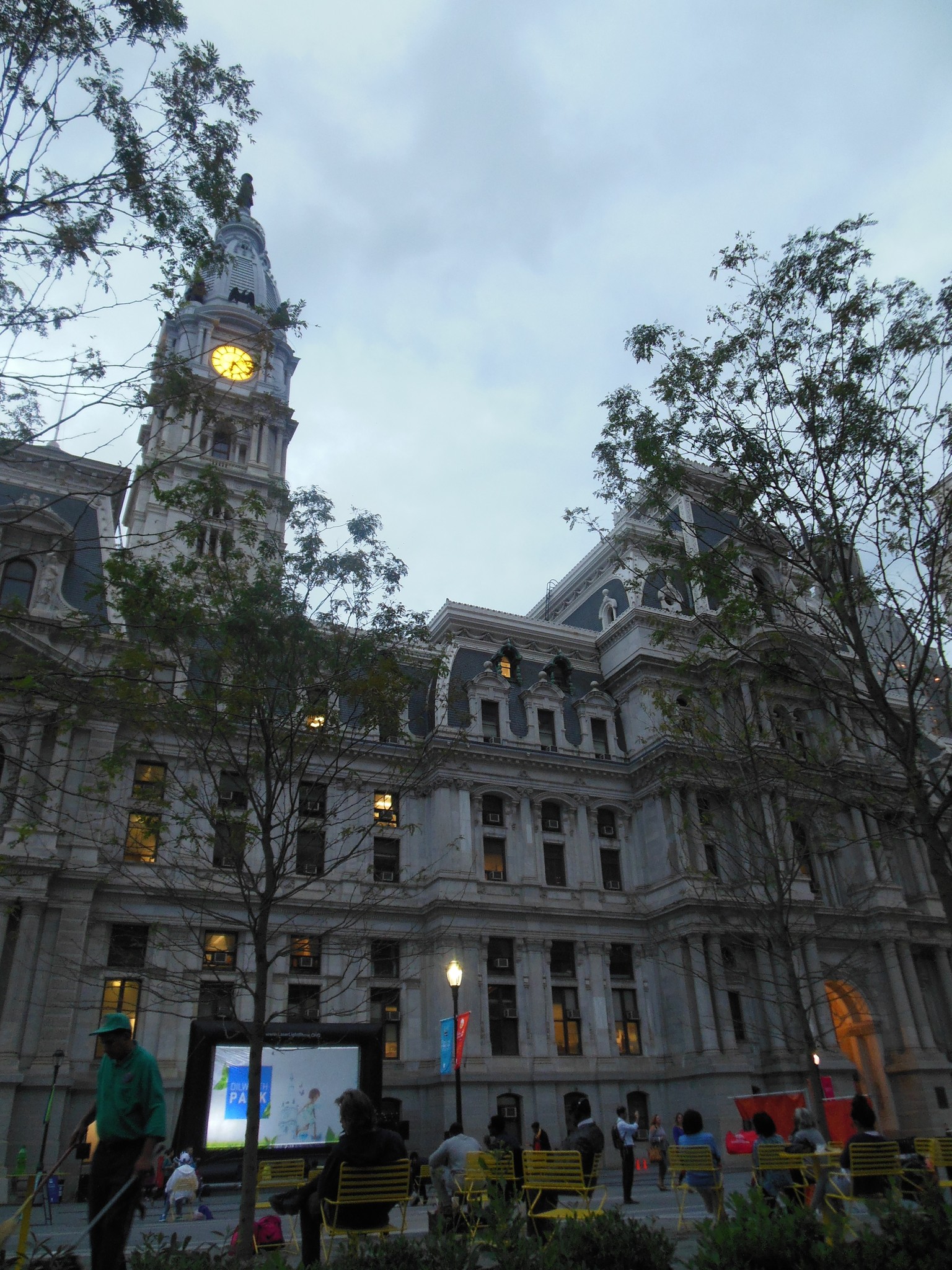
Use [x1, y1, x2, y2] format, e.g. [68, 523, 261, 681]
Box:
[611, 1120, 627, 1149]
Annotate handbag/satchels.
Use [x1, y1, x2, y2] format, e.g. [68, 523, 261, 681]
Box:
[647, 1148, 662, 1162]
[227, 1215, 286, 1256]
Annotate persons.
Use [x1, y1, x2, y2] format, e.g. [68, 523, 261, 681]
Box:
[130, 1087, 897, 1269]
[67, 1013, 166, 1270]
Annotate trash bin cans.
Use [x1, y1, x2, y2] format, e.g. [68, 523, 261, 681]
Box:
[58, 1179, 66, 1203]
[893, 1136, 939, 1202]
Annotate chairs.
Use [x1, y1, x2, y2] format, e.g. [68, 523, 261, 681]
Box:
[667, 1147, 731, 1235]
[169, 1178, 198, 1222]
[751, 1136, 952, 1246]
[414, 1165, 438, 1207]
[450, 1151, 521, 1249]
[523, 1150, 608, 1253]
[254, 1158, 305, 1254]
[320, 1160, 413, 1264]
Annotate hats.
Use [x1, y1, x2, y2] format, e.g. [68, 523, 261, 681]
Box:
[89, 1011, 131, 1034]
[180, 1153, 190, 1162]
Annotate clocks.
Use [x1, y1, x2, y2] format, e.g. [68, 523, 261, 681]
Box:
[209, 345, 259, 383]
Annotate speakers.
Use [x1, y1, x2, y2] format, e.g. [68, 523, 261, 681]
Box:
[76, 1143, 91, 1159]
[397, 1120, 410, 1137]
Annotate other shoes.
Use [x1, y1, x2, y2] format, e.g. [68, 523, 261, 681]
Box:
[159, 1215, 166, 1222]
[175, 1215, 182, 1221]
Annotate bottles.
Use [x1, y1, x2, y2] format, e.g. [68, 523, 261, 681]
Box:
[15, 1145, 28, 1175]
